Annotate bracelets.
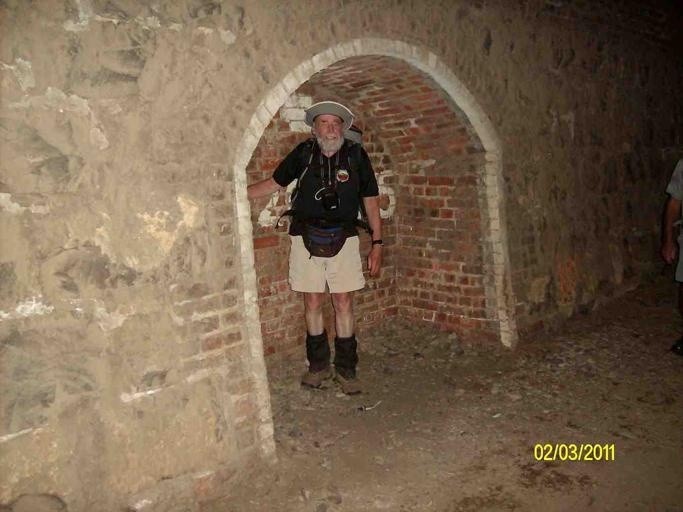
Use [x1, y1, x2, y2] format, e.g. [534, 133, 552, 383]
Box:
[372, 240, 384, 247]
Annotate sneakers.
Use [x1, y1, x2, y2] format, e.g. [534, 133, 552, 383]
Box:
[333, 364, 366, 396]
[299, 365, 334, 387]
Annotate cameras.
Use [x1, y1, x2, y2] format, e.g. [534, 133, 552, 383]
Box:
[322, 188, 340, 210]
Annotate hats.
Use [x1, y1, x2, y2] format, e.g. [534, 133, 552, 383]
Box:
[301, 99, 355, 131]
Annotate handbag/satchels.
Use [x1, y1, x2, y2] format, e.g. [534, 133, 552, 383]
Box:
[297, 223, 350, 258]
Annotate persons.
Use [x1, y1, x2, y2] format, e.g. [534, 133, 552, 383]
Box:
[248, 101, 385, 394]
[660, 157, 683, 356]
